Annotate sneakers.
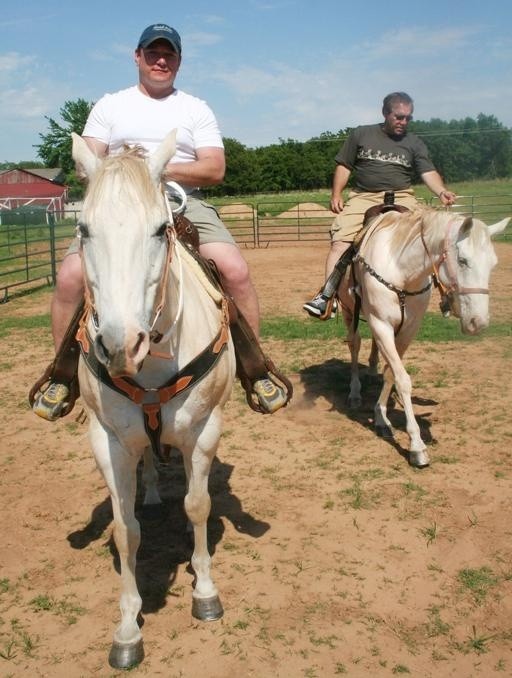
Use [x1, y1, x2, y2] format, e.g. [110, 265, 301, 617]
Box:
[249, 372, 287, 414]
[302, 293, 338, 318]
[33, 381, 69, 422]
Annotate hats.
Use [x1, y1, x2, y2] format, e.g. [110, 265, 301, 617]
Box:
[136, 23, 182, 55]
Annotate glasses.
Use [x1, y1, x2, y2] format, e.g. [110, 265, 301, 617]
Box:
[394, 114, 412, 120]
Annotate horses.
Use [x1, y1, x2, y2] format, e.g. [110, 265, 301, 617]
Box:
[71, 128, 237, 670]
[337, 205, 511, 467]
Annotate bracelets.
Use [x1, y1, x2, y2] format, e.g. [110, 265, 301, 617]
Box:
[438, 191, 445, 198]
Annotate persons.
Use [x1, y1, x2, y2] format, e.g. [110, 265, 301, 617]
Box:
[303, 91, 455, 320]
[27, 24, 287, 422]
